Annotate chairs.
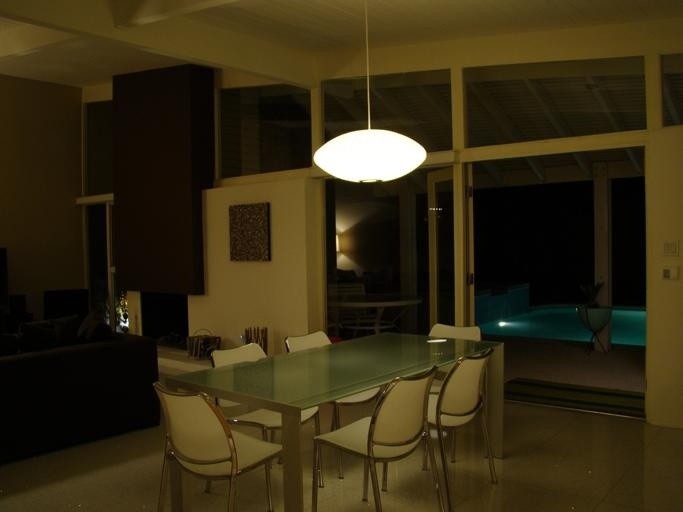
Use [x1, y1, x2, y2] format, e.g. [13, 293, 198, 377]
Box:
[210, 342, 326, 488]
[284, 328, 382, 479]
[312, 366, 446, 512]
[427, 348, 498, 512]
[153, 380, 283, 512]
[427, 324, 481, 394]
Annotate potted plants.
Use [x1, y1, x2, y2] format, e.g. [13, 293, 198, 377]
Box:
[577, 279, 606, 308]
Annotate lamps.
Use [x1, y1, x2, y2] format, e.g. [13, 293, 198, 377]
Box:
[312, 1, 428, 185]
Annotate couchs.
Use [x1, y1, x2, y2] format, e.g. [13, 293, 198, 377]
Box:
[1, 333, 160, 467]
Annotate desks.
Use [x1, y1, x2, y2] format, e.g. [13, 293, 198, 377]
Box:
[165, 331, 506, 512]
[323, 296, 420, 341]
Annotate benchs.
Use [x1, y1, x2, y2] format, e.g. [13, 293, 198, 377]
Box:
[328, 283, 378, 340]
